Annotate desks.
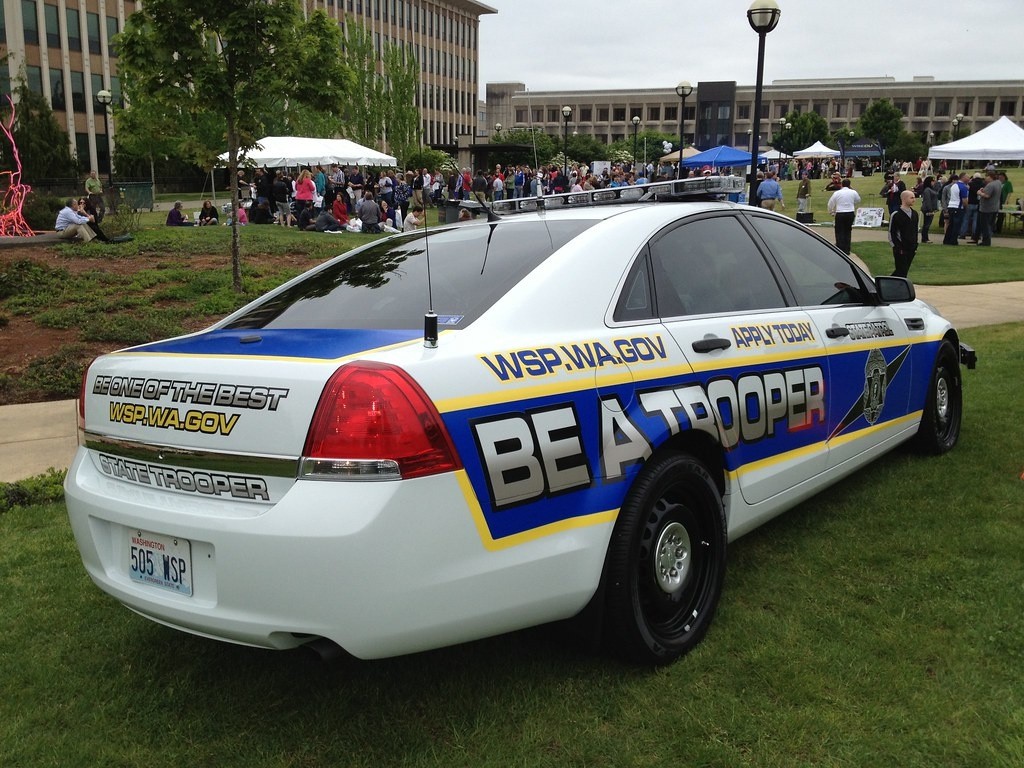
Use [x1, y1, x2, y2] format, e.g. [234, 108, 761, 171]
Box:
[996, 210, 1024, 233]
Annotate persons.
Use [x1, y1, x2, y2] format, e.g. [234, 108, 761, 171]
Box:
[943, 172, 969, 245]
[920, 176, 939, 242]
[227, 203, 247, 226]
[85, 170, 105, 222]
[55, 197, 113, 245]
[966, 170, 1001, 246]
[889, 190, 920, 279]
[795, 171, 811, 211]
[757, 171, 783, 211]
[238, 116, 1013, 246]
[166, 201, 197, 226]
[828, 180, 861, 255]
[199, 200, 219, 226]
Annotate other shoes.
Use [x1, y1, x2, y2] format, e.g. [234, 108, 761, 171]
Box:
[926, 241, 933, 243]
[960, 236, 965, 239]
[978, 242, 991, 246]
[967, 240, 976, 243]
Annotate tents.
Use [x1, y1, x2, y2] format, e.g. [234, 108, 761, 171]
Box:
[793, 140, 840, 175]
[843, 136, 884, 172]
[204, 136, 397, 200]
[760, 149, 792, 164]
[656, 146, 703, 177]
[683, 146, 766, 175]
[928, 116, 1024, 178]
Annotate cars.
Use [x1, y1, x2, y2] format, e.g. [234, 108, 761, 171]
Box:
[62, 176, 979, 671]
[860, 161, 872, 176]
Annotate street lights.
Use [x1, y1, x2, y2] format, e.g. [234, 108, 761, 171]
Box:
[850, 132, 855, 147]
[561, 105, 572, 176]
[950, 113, 964, 175]
[930, 133, 934, 143]
[779, 117, 792, 177]
[96, 90, 119, 215]
[632, 116, 640, 167]
[747, 129, 752, 152]
[746, 1, 782, 205]
[675, 82, 692, 179]
